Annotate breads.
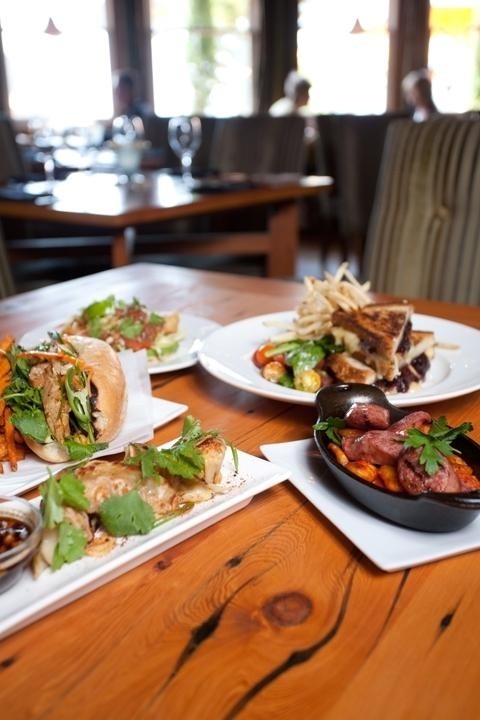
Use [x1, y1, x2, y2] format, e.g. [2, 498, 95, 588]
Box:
[323, 299, 436, 386]
[18, 332, 133, 464]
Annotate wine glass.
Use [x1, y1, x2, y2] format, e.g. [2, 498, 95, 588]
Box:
[33, 115, 203, 206]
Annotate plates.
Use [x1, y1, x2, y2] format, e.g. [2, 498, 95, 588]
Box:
[0, 432, 291, 636]
[195, 311, 479, 404]
[257, 437, 479, 575]
[2, 393, 188, 500]
[18, 312, 222, 377]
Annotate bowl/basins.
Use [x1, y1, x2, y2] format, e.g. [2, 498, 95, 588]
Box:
[314, 381, 480, 536]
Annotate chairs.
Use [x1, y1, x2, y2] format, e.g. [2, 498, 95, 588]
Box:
[189, 112, 382, 260]
[363, 111, 478, 307]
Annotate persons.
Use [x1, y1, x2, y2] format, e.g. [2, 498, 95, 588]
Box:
[267, 71, 319, 145]
[400, 68, 440, 122]
[100, 69, 144, 143]
